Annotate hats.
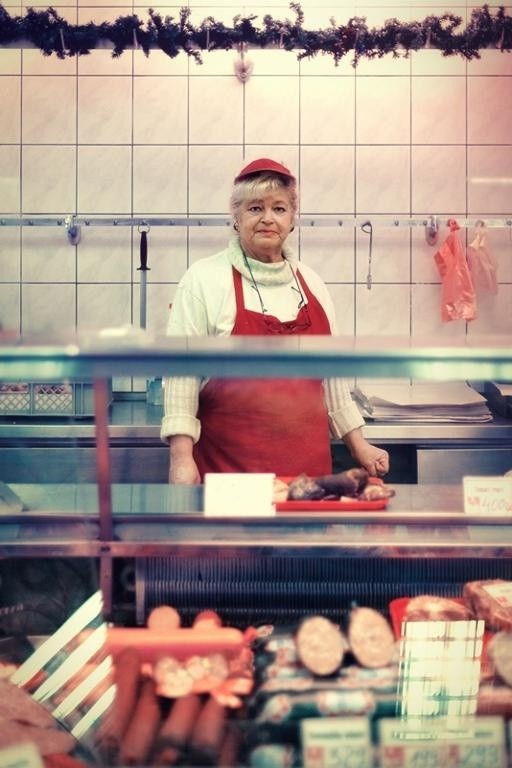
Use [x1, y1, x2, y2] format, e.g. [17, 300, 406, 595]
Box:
[233, 158, 294, 182]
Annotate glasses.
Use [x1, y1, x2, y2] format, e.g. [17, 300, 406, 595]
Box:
[251, 284, 313, 333]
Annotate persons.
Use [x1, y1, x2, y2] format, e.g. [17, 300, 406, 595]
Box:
[159, 158, 392, 485]
